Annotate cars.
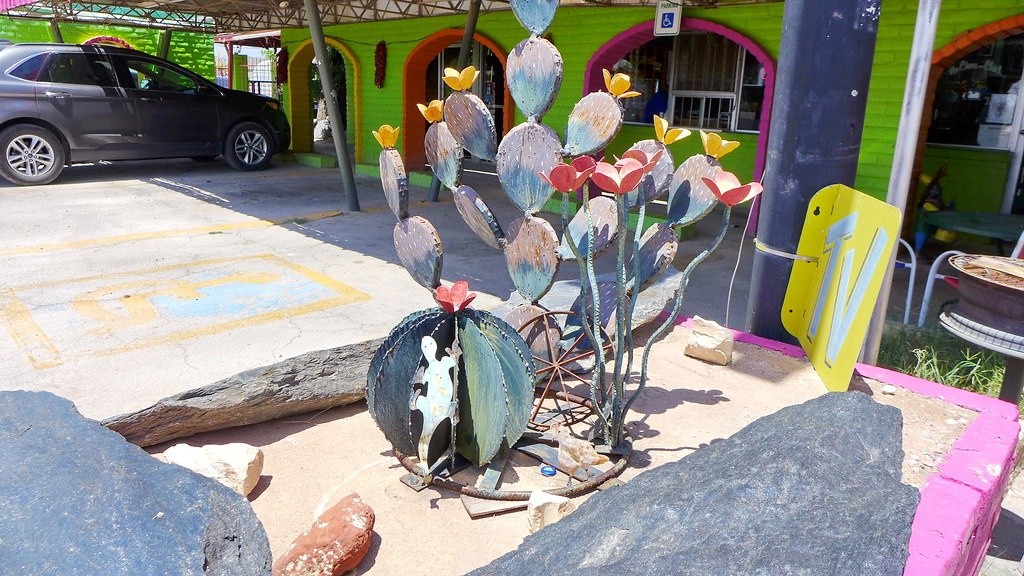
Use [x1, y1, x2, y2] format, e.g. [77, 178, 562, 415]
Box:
[0, 42, 293, 187]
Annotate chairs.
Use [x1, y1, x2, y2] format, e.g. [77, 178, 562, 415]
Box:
[92, 63, 114, 87]
[917, 231, 1024, 328]
[895, 238, 916, 325]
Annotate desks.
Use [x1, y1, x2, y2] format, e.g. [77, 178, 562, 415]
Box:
[920, 210, 1024, 257]
